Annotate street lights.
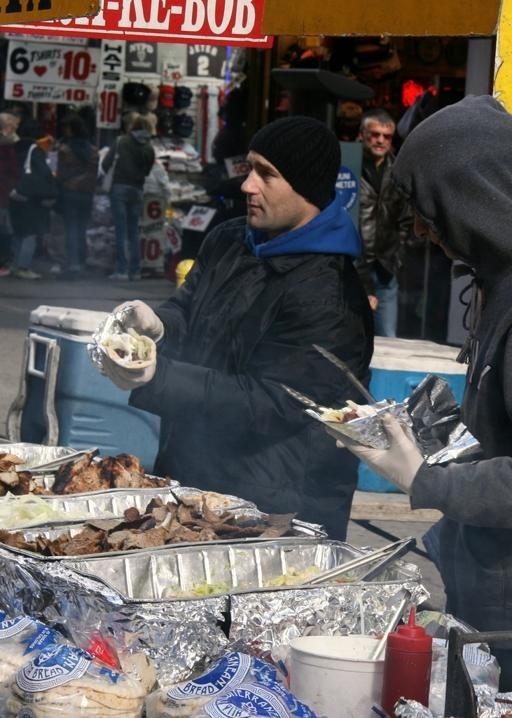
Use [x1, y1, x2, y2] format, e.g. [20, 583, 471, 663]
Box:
[15, 173, 56, 199]
[102, 170, 113, 191]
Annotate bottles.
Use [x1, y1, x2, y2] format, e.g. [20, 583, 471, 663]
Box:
[122, 81, 194, 137]
[248, 115, 342, 210]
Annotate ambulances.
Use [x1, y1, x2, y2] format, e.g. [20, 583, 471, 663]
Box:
[102, 328, 157, 370]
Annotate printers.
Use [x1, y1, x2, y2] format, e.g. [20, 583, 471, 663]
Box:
[281, 343, 380, 409]
[283, 536, 419, 584]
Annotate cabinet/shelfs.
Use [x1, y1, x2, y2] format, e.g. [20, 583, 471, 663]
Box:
[380, 605, 432, 714]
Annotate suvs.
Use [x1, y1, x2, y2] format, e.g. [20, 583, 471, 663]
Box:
[289, 635, 386, 715]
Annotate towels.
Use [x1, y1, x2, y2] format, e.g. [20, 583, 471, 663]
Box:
[359, 335, 472, 492]
[20, 305, 160, 474]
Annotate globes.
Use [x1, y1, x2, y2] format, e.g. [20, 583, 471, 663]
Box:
[0, 485, 298, 556]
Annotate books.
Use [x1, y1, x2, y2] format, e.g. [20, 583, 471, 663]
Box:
[359, 333, 468, 498]
[5, 304, 168, 472]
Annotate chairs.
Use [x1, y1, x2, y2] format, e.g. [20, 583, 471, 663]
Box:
[1, 264, 88, 279]
[109, 272, 141, 281]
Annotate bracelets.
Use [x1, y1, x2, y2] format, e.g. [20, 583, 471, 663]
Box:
[290, 633, 385, 718]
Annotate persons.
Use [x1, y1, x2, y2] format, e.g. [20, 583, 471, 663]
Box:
[331, 94, 512, 695]
[102, 110, 155, 281]
[353, 110, 414, 339]
[0, 101, 99, 282]
[332, 101, 364, 144]
[99, 114, 375, 544]
